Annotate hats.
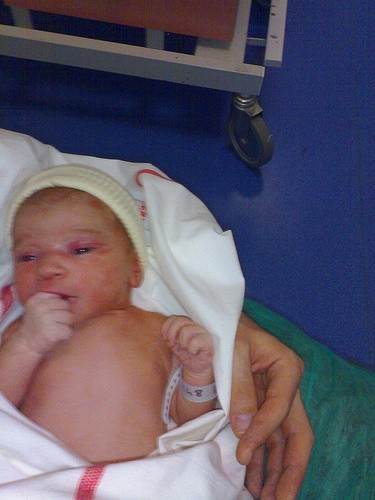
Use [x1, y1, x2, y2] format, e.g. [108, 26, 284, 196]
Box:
[7, 164, 148, 276]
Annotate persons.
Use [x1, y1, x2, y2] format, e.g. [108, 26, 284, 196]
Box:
[2, 126, 314, 500]
[0, 161, 215, 500]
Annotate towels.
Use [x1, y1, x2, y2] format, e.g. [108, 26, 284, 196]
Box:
[0, 126, 254, 500]
